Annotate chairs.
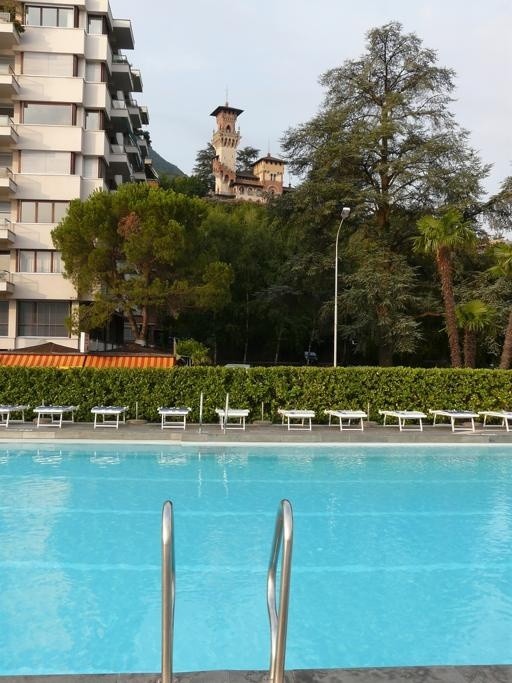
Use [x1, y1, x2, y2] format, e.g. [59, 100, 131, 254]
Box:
[278, 409, 315, 431]
[33, 405, 79, 429]
[477, 410, 512, 432]
[91, 406, 129, 429]
[324, 410, 367, 431]
[379, 409, 427, 432]
[216, 409, 249, 430]
[429, 408, 480, 433]
[0, 405, 30, 428]
[158, 406, 192, 431]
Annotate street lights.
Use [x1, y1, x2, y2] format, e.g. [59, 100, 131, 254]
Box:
[334, 207, 350, 367]
